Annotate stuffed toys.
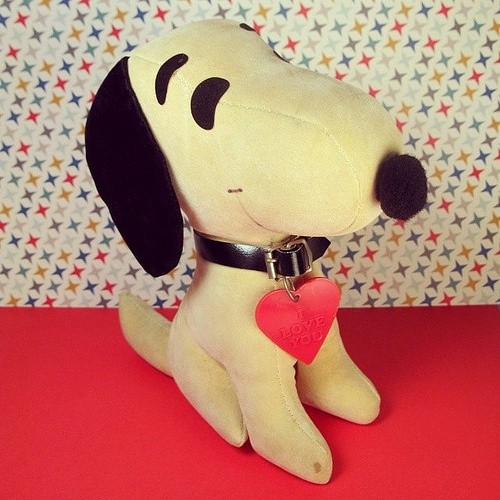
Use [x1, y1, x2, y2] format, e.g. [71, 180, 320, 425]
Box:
[84, 18, 429, 484]
